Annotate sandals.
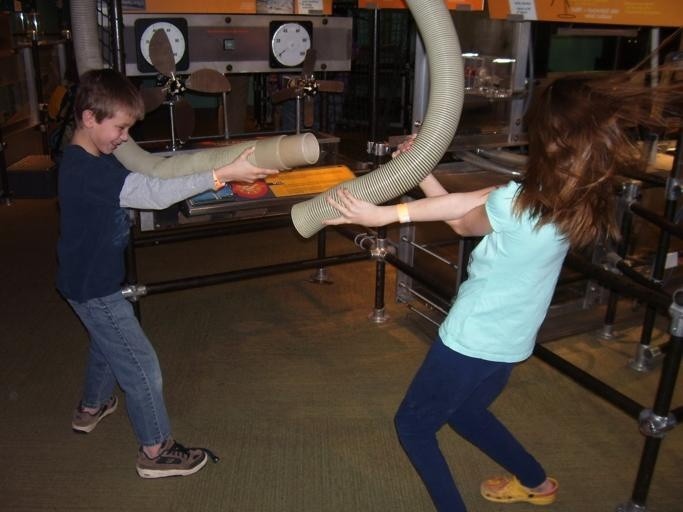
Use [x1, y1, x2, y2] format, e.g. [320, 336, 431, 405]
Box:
[481, 476, 559, 506]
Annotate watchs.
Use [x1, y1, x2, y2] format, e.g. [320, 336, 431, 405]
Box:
[213, 171, 225, 190]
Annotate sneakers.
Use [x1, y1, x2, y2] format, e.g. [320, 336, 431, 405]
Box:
[136, 435, 208, 479]
[71, 394, 119, 433]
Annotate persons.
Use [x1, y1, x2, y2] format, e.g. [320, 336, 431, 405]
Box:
[321, 28, 683, 512]
[57, 70, 280, 480]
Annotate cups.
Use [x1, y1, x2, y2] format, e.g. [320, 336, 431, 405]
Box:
[367, 140, 388, 157]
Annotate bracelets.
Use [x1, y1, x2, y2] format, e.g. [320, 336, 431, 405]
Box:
[397, 204, 411, 224]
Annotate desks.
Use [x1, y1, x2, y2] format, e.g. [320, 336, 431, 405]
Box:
[128, 149, 396, 329]
